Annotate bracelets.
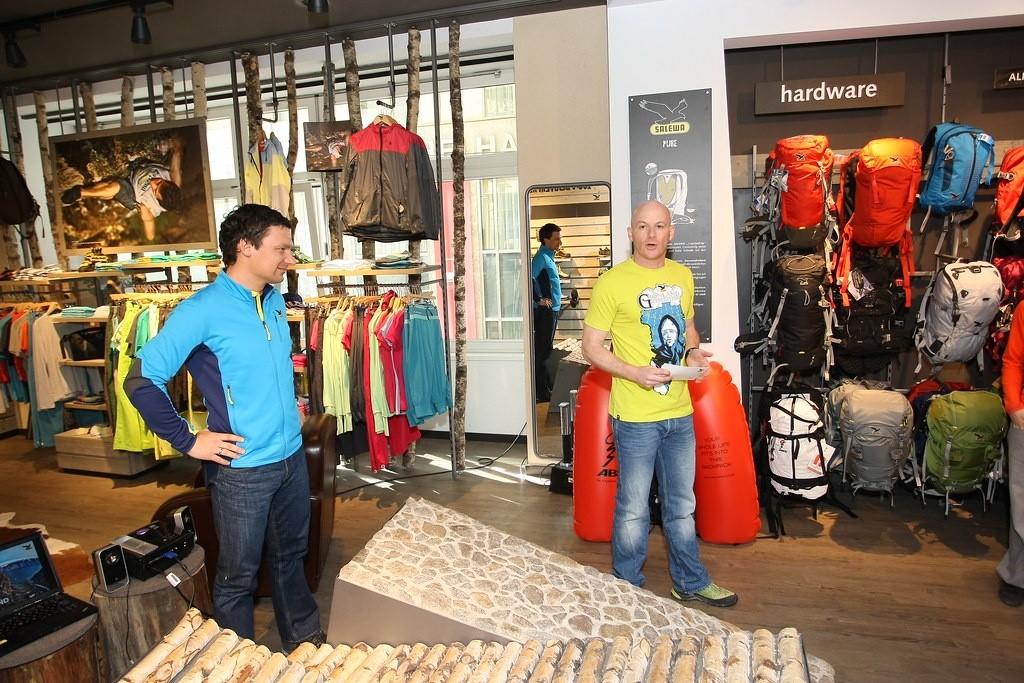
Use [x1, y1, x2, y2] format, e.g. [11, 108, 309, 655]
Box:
[685, 348, 696, 360]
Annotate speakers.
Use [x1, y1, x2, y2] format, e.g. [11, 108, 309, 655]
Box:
[168, 506, 197, 543]
[92, 542, 129, 594]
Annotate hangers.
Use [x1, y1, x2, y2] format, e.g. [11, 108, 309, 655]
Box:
[257, 119, 268, 146]
[270, 121, 275, 140]
[374, 101, 398, 126]
[118, 285, 195, 304]
[303, 282, 436, 319]
[0, 291, 62, 315]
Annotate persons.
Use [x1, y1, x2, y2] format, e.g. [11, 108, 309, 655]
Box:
[532, 223, 563, 402]
[582, 200, 739, 609]
[994, 300, 1024, 606]
[122, 203, 327, 658]
[63, 157, 182, 241]
[305, 130, 351, 167]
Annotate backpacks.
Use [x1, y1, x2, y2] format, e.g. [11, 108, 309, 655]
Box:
[753, 379, 1009, 505]
[831, 255, 911, 373]
[836, 136, 922, 246]
[987, 256, 1024, 363]
[914, 259, 1002, 364]
[759, 254, 831, 371]
[994, 145, 1024, 258]
[921, 123, 994, 215]
[763, 135, 833, 249]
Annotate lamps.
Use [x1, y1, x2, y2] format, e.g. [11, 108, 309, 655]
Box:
[129, 3, 152, 45]
[302, 0, 329, 13]
[3, 31, 28, 69]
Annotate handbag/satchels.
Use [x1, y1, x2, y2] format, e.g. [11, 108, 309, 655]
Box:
[59, 326, 106, 362]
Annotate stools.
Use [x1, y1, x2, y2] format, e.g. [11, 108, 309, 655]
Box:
[0, 612, 110, 683]
[89, 541, 214, 683]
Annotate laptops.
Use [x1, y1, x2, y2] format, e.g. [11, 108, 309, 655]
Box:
[0, 531, 100, 658]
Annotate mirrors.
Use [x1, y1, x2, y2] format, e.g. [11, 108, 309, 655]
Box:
[525, 180, 614, 467]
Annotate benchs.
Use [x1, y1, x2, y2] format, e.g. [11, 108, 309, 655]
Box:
[54, 426, 170, 476]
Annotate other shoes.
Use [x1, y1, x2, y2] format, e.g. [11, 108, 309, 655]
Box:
[999, 580, 1024, 606]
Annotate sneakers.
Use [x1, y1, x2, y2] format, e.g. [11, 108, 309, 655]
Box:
[536, 385, 553, 401]
[670, 581, 738, 607]
[281, 631, 327, 656]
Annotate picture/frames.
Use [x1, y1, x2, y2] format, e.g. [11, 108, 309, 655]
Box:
[49, 116, 219, 256]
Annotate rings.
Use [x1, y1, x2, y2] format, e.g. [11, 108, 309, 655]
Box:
[219, 448, 223, 455]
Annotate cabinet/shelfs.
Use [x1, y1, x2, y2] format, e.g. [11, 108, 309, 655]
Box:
[553, 258, 571, 305]
[287, 315, 309, 395]
[599, 256, 611, 277]
[0, 258, 442, 301]
[53, 317, 108, 430]
